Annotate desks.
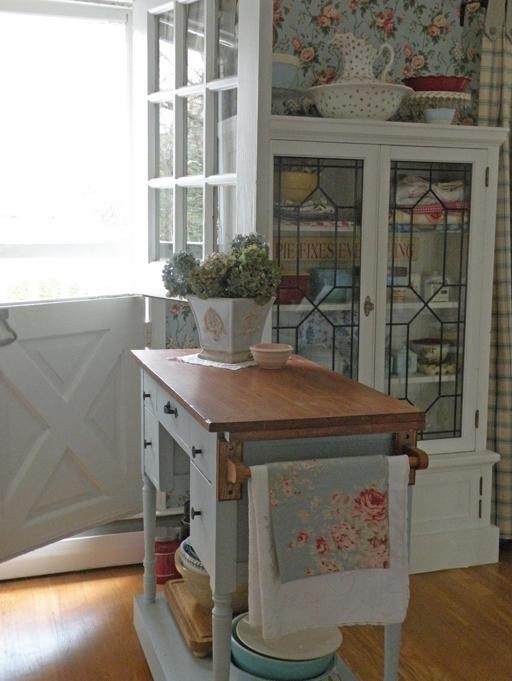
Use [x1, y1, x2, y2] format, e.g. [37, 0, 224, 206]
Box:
[128, 348, 429, 680]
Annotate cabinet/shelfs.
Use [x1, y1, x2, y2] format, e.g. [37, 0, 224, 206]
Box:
[213, 114, 508, 574]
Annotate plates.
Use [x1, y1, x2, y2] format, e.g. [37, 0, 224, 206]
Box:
[403, 74, 473, 92]
[236, 611, 343, 660]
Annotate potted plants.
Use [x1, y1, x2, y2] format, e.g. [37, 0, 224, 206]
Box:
[162, 234, 282, 364]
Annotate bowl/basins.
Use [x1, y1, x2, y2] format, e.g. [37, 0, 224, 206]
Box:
[228, 613, 336, 681]
[411, 337, 451, 363]
[423, 107, 455, 123]
[249, 344, 294, 370]
[306, 82, 415, 120]
[173, 536, 249, 614]
[273, 171, 319, 206]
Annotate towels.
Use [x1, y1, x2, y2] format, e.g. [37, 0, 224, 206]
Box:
[244, 452, 412, 640]
